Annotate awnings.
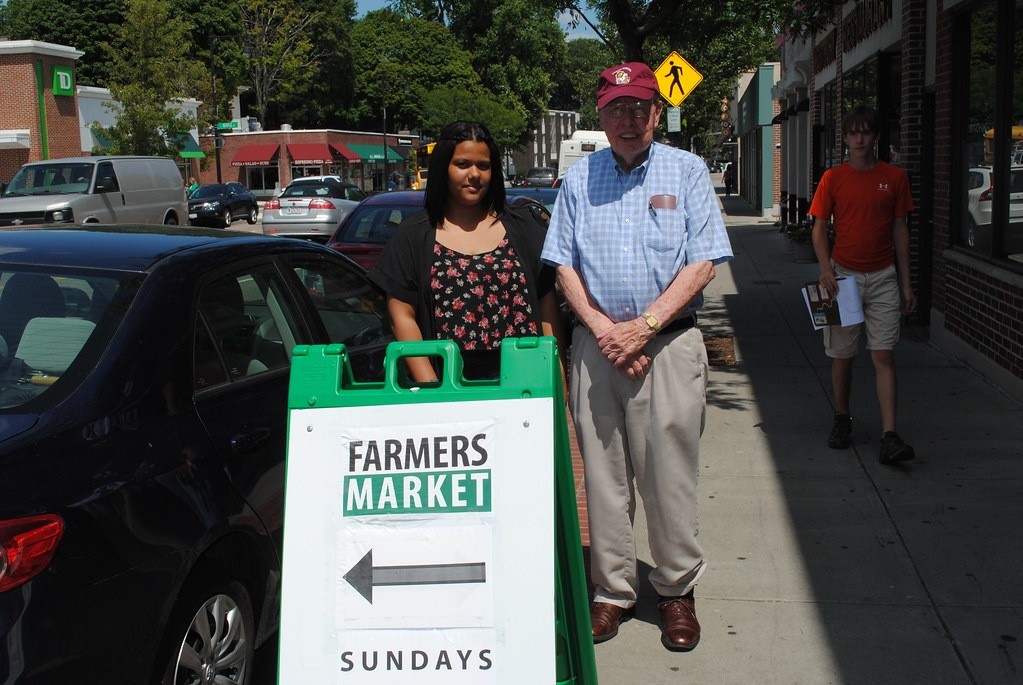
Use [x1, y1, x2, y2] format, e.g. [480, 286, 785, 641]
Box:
[286, 144, 333, 164]
[231, 144, 280, 165]
[346, 144, 404, 163]
[90, 127, 173, 156]
[168, 132, 207, 158]
[328, 142, 361, 163]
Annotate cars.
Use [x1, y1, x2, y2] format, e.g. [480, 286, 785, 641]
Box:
[328, 190, 427, 269]
[0, 224, 418, 685]
[505, 189, 561, 230]
[262, 183, 367, 241]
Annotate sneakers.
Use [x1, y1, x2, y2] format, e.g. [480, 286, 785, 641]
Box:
[828, 411, 853, 449]
[879, 431, 915, 465]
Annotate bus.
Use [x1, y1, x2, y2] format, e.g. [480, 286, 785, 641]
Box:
[558, 130, 611, 181]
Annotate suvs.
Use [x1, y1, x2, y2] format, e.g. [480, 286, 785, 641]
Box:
[524, 167, 557, 187]
[277, 176, 347, 198]
[965, 166, 1023, 248]
[188, 181, 260, 227]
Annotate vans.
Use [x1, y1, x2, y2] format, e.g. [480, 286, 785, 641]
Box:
[0, 155, 189, 228]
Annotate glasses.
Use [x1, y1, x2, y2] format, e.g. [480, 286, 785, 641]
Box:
[601, 97, 659, 118]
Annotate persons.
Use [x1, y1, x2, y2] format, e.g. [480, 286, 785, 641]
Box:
[540, 61, 736, 652]
[808, 103, 917, 467]
[721, 166, 734, 197]
[365, 119, 569, 411]
[183, 177, 199, 195]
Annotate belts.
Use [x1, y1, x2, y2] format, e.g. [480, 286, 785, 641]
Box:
[579, 313, 700, 336]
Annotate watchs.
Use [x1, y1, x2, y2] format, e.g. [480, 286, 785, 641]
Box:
[641, 310, 662, 333]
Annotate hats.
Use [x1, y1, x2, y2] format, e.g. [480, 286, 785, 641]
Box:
[597, 62, 660, 109]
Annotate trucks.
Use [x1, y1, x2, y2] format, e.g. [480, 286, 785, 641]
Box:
[411, 142, 436, 190]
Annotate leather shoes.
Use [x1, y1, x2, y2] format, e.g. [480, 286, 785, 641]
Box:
[657, 591, 701, 651]
[590, 602, 636, 643]
